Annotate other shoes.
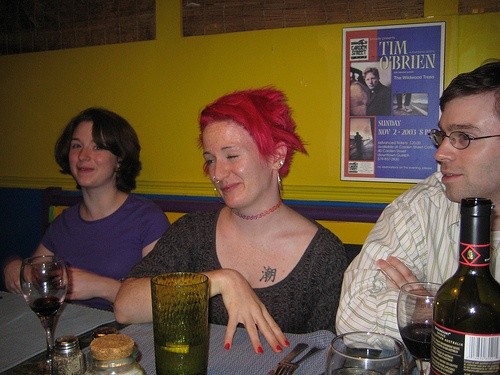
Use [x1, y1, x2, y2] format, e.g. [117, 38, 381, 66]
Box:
[405, 107, 412, 113]
[397, 106, 402, 111]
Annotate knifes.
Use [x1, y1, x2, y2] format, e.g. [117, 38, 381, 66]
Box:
[266, 343, 309, 375]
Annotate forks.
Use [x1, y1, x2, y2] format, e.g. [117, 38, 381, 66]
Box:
[274, 346, 326, 375]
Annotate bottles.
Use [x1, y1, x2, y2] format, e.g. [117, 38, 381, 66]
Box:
[429, 196, 500, 375]
[81, 333, 147, 375]
[51, 335, 87, 375]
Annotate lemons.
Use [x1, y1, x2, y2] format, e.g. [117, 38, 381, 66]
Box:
[163, 344, 189, 353]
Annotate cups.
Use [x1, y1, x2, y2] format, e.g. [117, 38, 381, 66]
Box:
[324, 331, 409, 375]
[149, 271, 210, 374]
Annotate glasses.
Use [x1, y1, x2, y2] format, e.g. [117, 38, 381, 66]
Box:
[428, 129, 500, 150]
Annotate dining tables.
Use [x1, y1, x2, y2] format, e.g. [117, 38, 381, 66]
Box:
[0, 291, 422, 375]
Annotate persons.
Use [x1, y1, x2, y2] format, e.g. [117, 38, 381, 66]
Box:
[114, 88, 349, 353]
[354, 67, 412, 150]
[335, 61, 500, 349]
[0, 107, 171, 312]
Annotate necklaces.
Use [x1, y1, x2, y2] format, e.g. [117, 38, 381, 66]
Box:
[231, 198, 281, 220]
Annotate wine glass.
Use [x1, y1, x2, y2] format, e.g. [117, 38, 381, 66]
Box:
[395, 282, 443, 375]
[20, 254, 69, 375]
